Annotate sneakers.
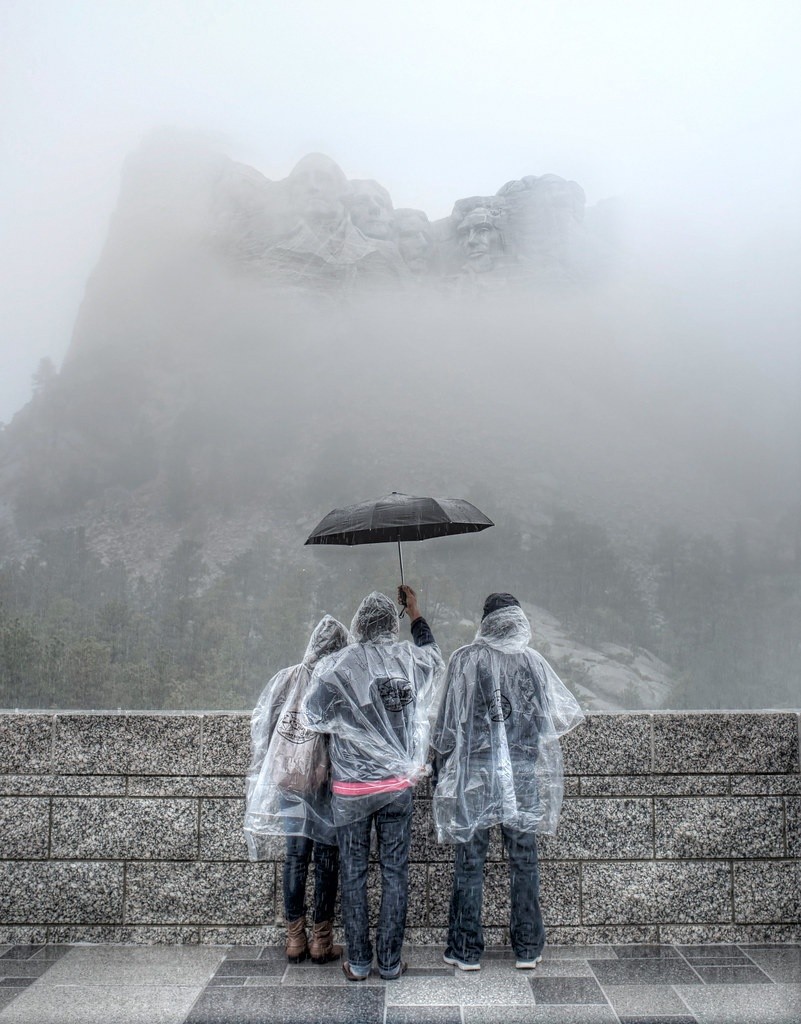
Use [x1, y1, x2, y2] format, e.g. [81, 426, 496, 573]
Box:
[443, 947, 481, 970]
[515, 953, 542, 968]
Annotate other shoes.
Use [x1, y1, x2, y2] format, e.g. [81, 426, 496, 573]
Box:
[342, 961, 366, 980]
[380, 960, 408, 979]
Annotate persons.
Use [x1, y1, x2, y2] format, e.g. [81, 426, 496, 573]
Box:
[432, 593, 583, 969]
[244, 614, 349, 964]
[301, 586, 445, 980]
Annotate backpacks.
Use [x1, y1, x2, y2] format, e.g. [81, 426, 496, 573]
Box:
[276, 665, 329, 793]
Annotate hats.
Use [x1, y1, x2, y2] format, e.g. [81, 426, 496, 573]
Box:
[481, 592, 521, 636]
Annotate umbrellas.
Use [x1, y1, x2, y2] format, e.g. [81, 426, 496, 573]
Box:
[303, 492, 495, 605]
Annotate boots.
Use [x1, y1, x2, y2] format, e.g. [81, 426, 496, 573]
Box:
[286, 915, 311, 964]
[309, 919, 343, 965]
